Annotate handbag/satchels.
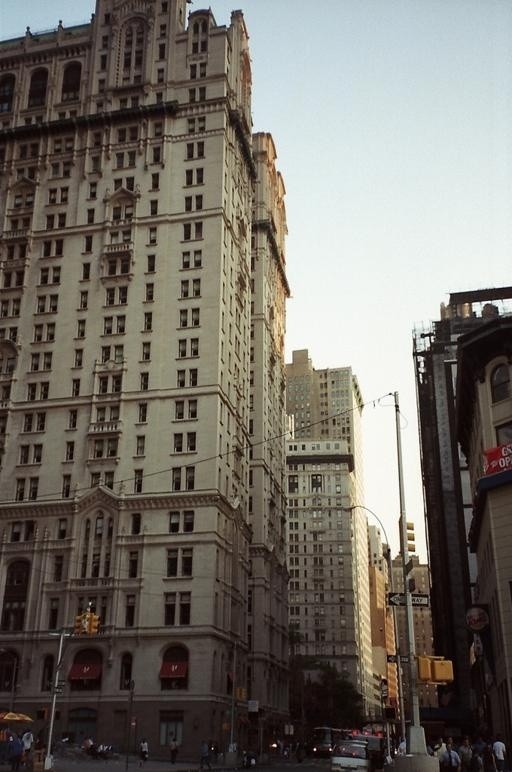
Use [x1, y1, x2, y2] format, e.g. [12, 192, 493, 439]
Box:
[145, 754, 148, 758]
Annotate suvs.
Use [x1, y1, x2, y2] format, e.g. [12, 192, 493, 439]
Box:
[353, 734, 385, 769]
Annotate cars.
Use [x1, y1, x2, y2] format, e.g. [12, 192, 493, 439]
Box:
[383, 737, 395, 759]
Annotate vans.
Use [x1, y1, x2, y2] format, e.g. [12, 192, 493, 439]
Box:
[328, 739, 372, 772]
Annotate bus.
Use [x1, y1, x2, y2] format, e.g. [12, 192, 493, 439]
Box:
[310, 726, 353, 758]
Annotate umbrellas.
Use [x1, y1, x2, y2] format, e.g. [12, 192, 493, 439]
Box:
[0, 711, 34, 732]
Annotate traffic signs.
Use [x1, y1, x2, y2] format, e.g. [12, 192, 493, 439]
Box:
[388, 593, 429, 608]
[387, 656, 409, 663]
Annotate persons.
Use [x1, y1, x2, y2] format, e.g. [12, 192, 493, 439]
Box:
[398, 736, 507, 772]
[0, 727, 237, 772]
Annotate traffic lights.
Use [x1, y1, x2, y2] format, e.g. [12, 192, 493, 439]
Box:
[72, 611, 101, 636]
[405, 522, 416, 554]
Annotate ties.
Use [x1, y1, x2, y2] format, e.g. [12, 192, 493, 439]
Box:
[449, 752, 452, 766]
